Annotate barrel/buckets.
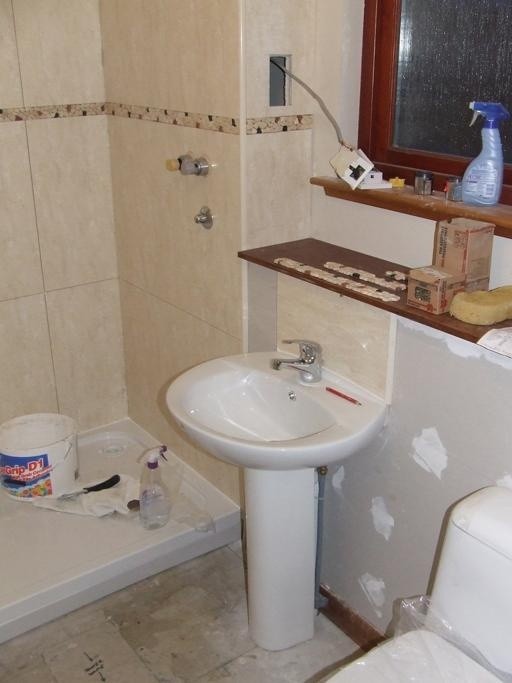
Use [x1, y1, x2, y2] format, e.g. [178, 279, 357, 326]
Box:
[1, 413, 80, 502]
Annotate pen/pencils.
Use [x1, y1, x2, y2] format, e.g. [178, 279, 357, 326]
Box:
[326, 386, 361, 406]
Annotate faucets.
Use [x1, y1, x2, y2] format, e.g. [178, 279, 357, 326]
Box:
[272, 339, 322, 383]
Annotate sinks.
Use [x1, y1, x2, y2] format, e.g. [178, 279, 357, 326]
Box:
[165, 350, 388, 470]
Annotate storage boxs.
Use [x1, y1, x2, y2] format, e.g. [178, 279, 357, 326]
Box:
[407, 265, 465, 318]
[432, 217, 496, 295]
